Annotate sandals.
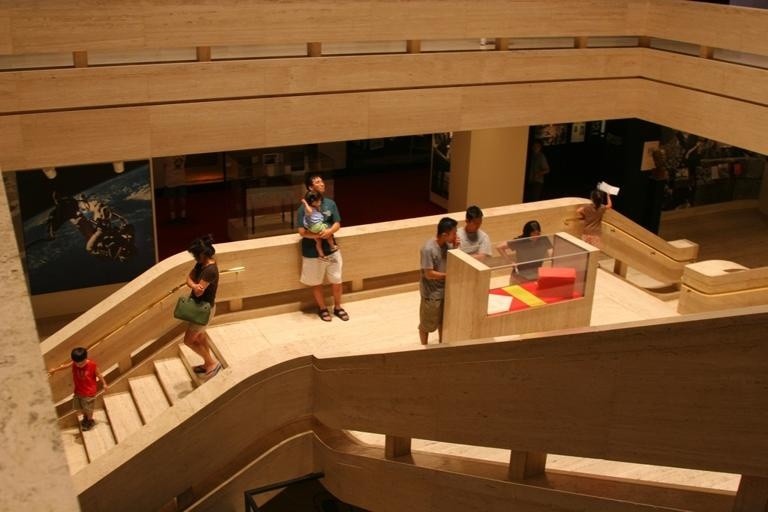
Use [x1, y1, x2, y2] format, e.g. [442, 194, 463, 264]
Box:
[320, 309, 330, 321]
[334, 307, 349, 321]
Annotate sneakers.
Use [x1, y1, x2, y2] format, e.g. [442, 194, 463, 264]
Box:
[193, 365, 206, 373]
[206, 361, 221, 379]
[81, 416, 94, 431]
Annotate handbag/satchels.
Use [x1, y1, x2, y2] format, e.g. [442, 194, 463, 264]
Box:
[174, 288, 211, 325]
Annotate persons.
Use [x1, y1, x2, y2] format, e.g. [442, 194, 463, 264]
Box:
[417, 217, 461, 345]
[293, 173, 349, 321]
[575, 191, 613, 266]
[46, 347, 110, 431]
[496, 220, 554, 285]
[183, 233, 221, 382]
[299, 189, 339, 264]
[456, 206, 493, 261]
[527, 140, 549, 202]
